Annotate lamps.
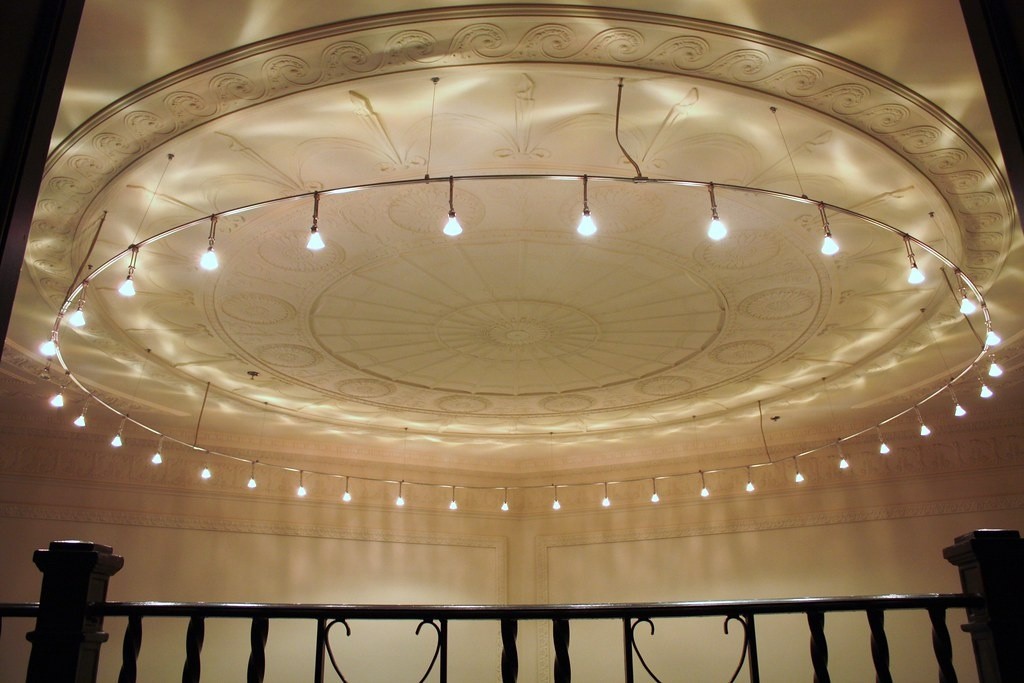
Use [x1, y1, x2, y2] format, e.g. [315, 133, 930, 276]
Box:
[984, 321, 1000, 346]
[818, 201, 839, 255]
[699, 472, 709, 496]
[836, 438, 849, 468]
[973, 363, 993, 398]
[876, 424, 890, 454]
[577, 174, 597, 236]
[450, 486, 457, 509]
[118, 246, 139, 296]
[553, 485, 560, 509]
[199, 214, 218, 269]
[396, 482, 404, 505]
[306, 189, 325, 251]
[746, 466, 755, 492]
[707, 180, 727, 240]
[68, 281, 88, 326]
[73, 395, 92, 427]
[299, 470, 306, 496]
[954, 269, 976, 314]
[38, 331, 56, 356]
[651, 479, 660, 503]
[50, 371, 70, 407]
[913, 403, 931, 436]
[201, 451, 211, 478]
[151, 435, 163, 463]
[948, 384, 966, 416]
[500, 488, 509, 511]
[110, 417, 126, 446]
[987, 349, 1002, 377]
[902, 234, 924, 284]
[601, 482, 610, 506]
[443, 175, 462, 236]
[791, 455, 804, 483]
[342, 476, 351, 501]
[248, 461, 256, 488]
